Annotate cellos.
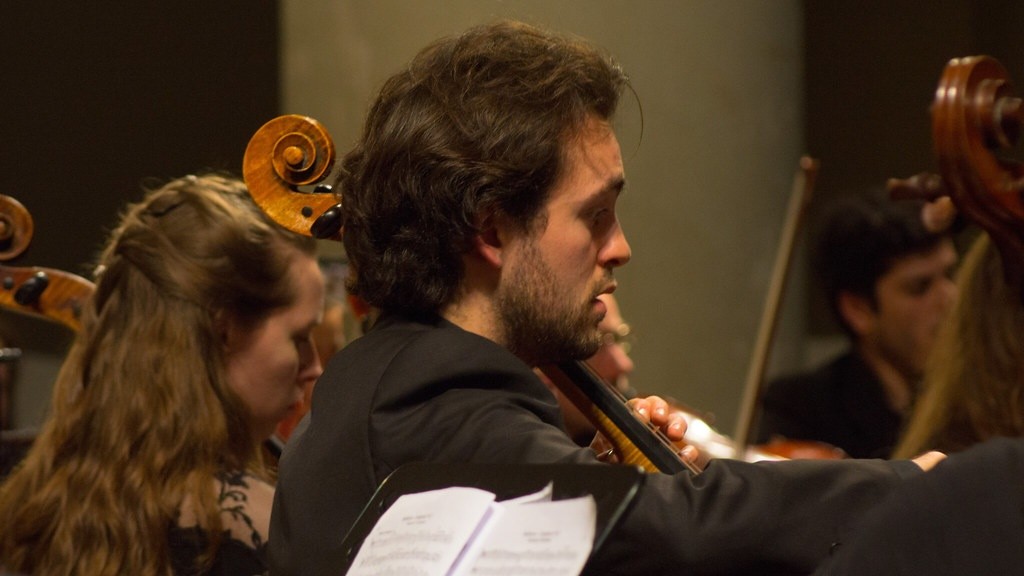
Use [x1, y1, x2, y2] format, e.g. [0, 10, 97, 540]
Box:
[873, 40, 1024, 233]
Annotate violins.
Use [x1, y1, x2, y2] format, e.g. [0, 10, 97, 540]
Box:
[239, 112, 706, 485]
[0, 189, 96, 339]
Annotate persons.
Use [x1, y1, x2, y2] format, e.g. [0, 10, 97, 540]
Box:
[531, 289, 783, 462]
[0, 176, 325, 576]
[263, 16, 1024, 576]
[894, 232, 1024, 461]
[757, 202, 957, 462]
